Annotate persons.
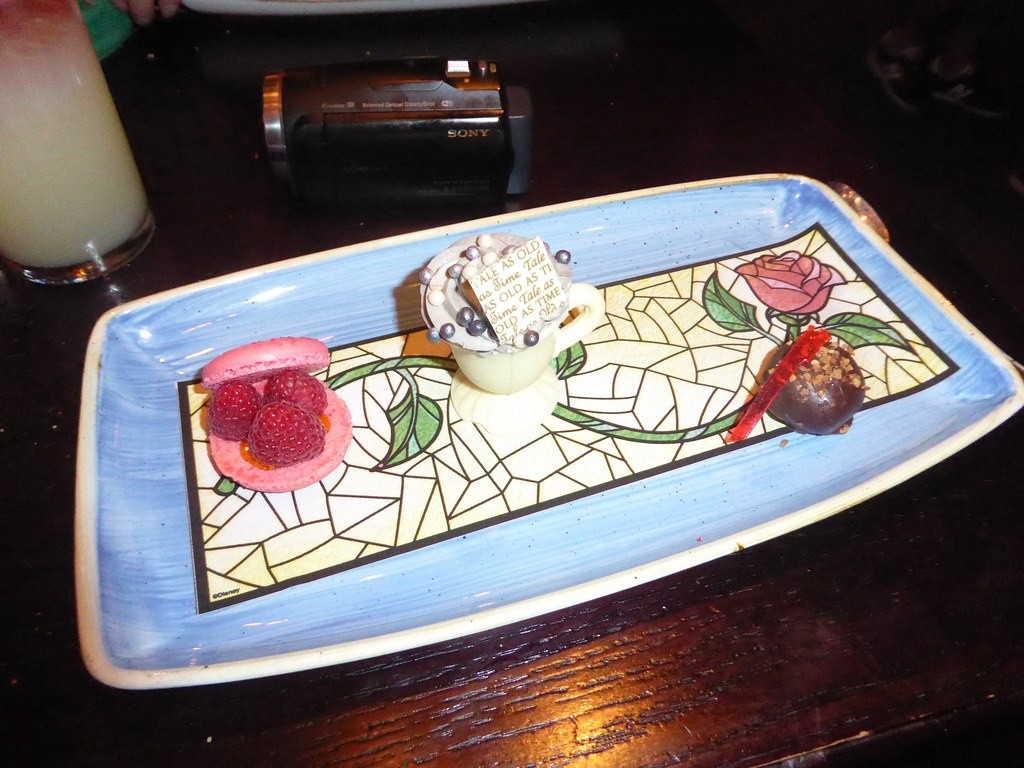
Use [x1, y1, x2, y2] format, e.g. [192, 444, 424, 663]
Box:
[78, 0, 181, 64]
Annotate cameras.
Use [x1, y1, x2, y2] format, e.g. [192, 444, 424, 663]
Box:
[260, 59, 532, 214]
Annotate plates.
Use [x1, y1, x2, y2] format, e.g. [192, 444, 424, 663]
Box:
[74, 173, 1023, 690]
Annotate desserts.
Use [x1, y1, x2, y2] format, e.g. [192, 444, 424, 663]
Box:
[727, 327, 866, 442]
[418, 232, 605, 394]
[199, 337, 354, 492]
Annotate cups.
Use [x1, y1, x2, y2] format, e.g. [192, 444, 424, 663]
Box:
[0, 0, 156, 284]
[450, 283, 605, 394]
